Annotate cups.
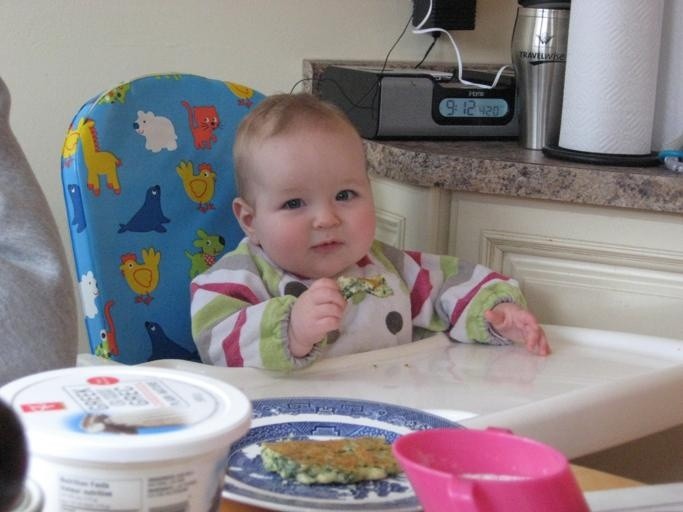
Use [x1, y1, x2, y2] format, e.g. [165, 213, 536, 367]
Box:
[394, 419, 594, 511]
[509, 5, 570, 150]
[0, 366, 253, 511]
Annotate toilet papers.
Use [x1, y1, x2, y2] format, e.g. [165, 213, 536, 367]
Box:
[557, 0, 683, 155]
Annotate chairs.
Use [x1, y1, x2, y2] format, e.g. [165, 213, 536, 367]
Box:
[59, 73, 268, 366]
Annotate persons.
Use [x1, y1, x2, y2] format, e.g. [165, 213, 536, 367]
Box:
[0, 398, 27, 512]
[188, 89, 551, 374]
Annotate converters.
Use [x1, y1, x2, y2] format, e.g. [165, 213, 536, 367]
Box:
[412, 0, 476, 37]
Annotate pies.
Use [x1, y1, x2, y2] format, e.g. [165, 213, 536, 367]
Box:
[261, 437, 406, 485]
[338, 275, 394, 300]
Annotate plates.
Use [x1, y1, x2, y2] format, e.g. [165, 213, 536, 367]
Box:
[216, 394, 461, 508]
